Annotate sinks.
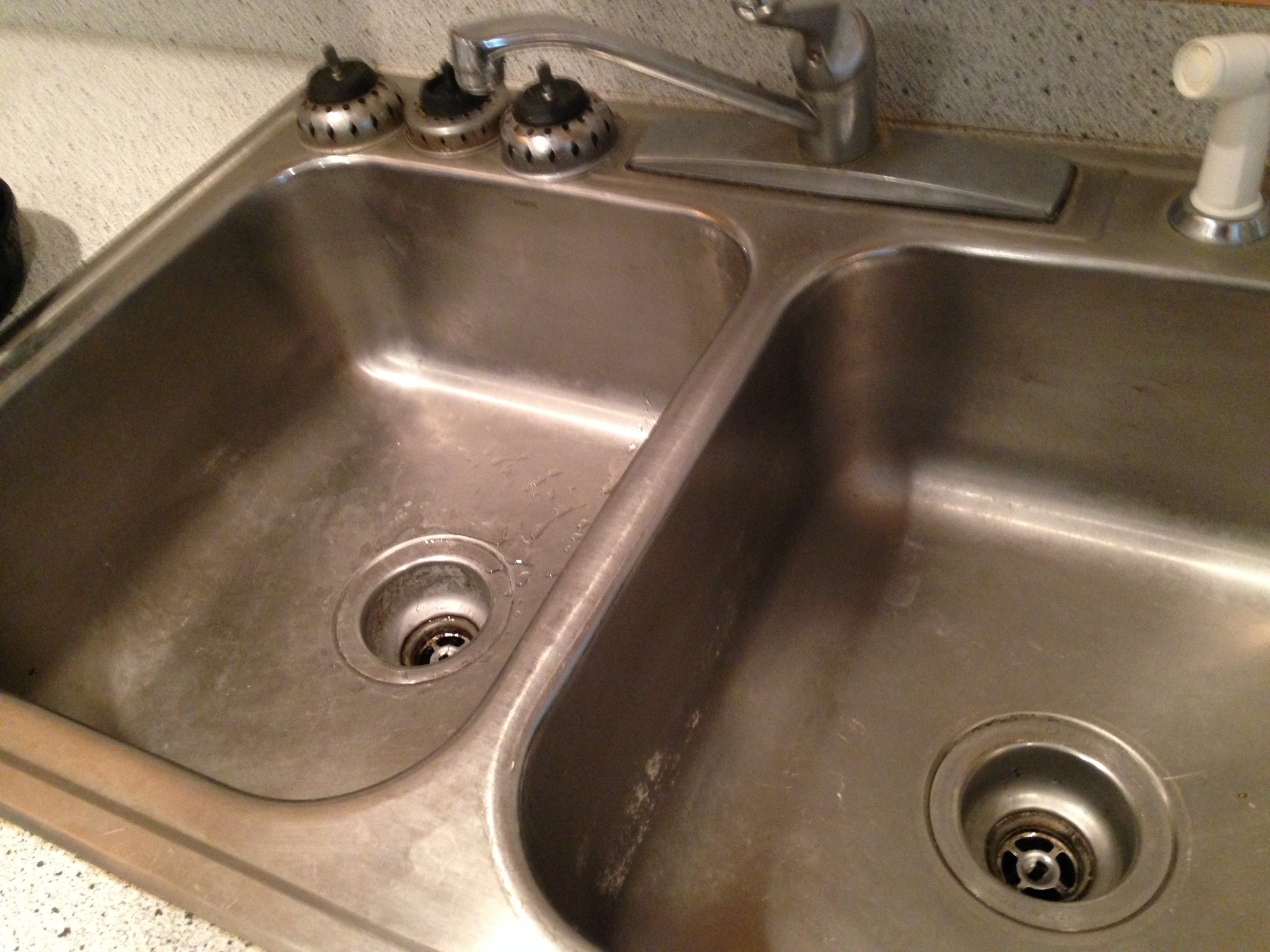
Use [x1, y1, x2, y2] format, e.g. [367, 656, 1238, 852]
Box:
[481, 111, 1270, 952]
[2, 64, 774, 949]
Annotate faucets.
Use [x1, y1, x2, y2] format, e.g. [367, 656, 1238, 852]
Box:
[451, 1, 880, 166]
